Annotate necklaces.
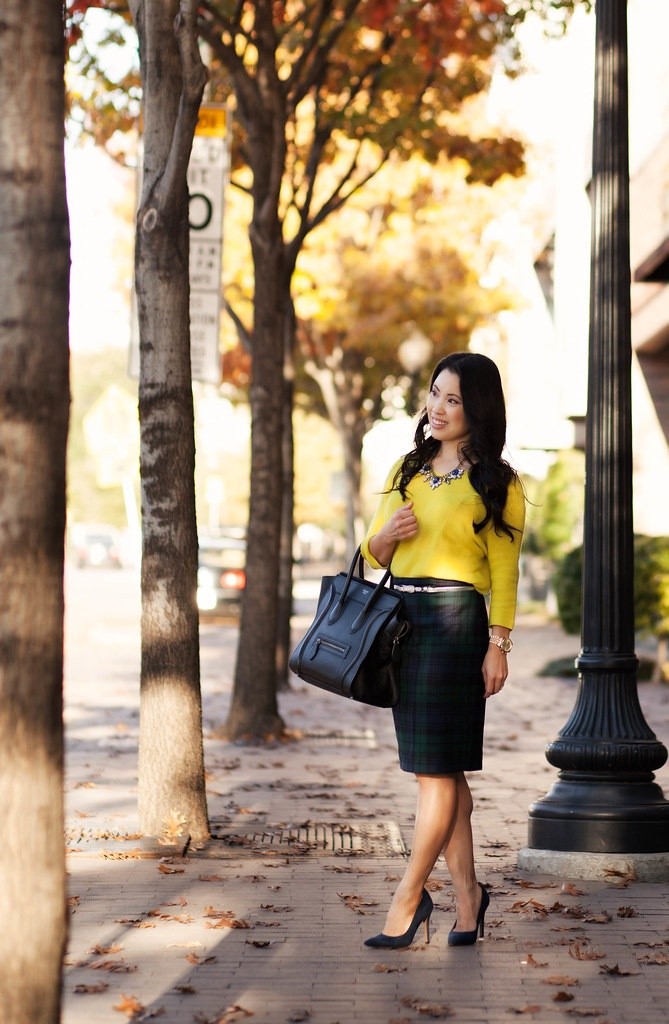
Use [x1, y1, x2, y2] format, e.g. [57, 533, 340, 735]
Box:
[419, 442, 472, 491]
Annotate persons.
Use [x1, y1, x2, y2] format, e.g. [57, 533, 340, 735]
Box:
[361, 353, 525, 944]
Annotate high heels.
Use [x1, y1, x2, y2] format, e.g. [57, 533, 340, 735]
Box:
[364, 887, 433, 948]
[448, 881, 490, 947]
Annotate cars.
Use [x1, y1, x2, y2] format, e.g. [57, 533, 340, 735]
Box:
[196, 528, 248, 611]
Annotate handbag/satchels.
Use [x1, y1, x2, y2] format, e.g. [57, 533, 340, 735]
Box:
[288, 543, 410, 709]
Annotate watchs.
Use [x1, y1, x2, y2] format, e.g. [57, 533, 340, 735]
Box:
[489, 634, 513, 653]
